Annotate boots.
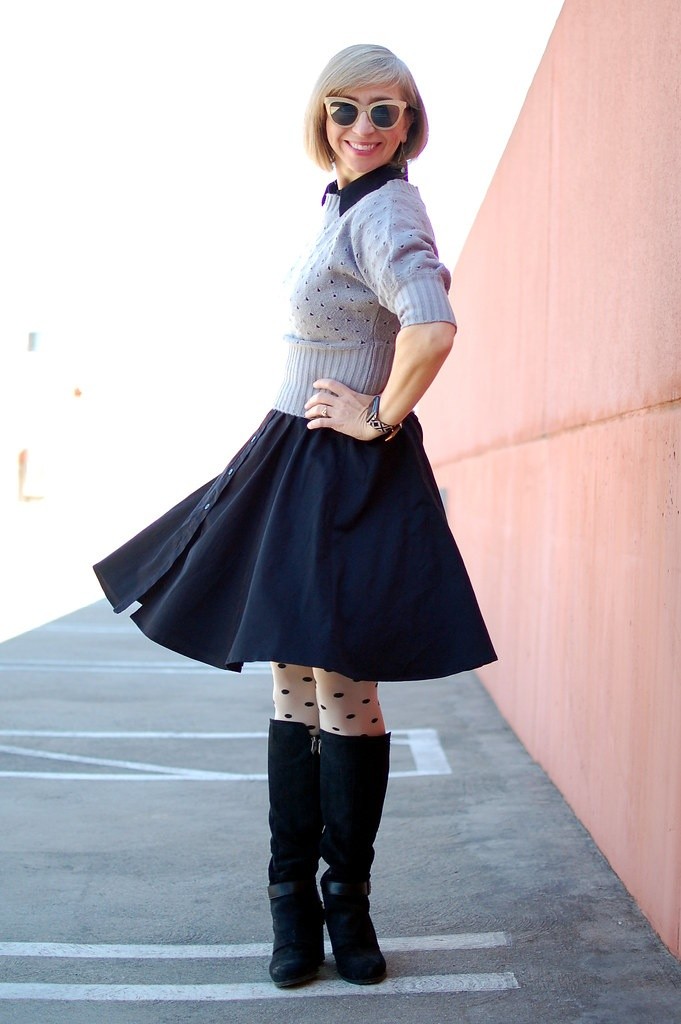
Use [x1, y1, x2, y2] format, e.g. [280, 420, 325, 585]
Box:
[268, 718, 324, 988]
[319, 728, 388, 984]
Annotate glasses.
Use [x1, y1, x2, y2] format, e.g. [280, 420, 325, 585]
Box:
[323, 96, 406, 130]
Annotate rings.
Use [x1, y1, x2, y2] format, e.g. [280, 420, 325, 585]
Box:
[322, 405, 327, 417]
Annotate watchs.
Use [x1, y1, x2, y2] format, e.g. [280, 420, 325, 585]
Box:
[366, 394, 403, 441]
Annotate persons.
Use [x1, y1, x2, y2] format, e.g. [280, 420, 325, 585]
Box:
[96, 45, 500, 986]
